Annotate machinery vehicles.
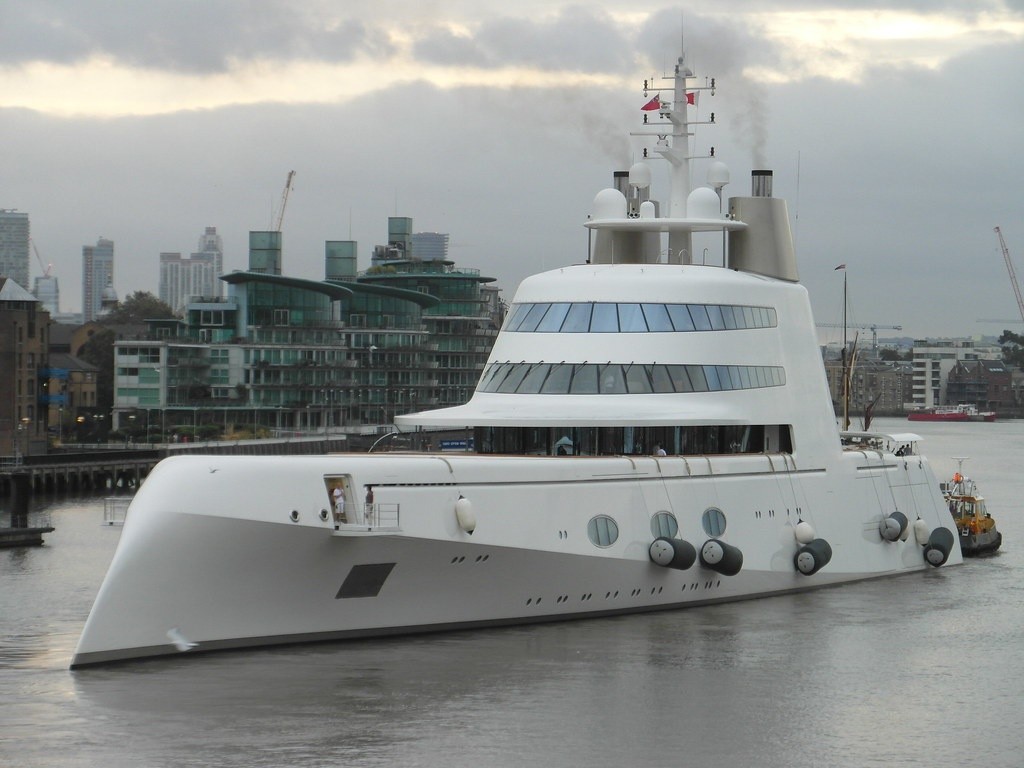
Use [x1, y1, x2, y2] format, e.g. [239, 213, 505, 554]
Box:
[992, 223, 1023, 322]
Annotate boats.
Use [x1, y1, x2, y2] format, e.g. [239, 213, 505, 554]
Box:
[68, 19, 964, 667]
[907, 403, 996, 422]
[884, 432, 923, 456]
[939, 457, 1003, 557]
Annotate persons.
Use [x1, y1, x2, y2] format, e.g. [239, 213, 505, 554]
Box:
[897, 444, 911, 456]
[652, 441, 667, 455]
[333, 483, 346, 523]
[557, 446, 567, 455]
[365, 486, 373, 526]
[604, 371, 614, 393]
[173, 433, 177, 443]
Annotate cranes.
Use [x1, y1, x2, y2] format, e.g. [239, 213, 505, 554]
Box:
[815, 322, 903, 347]
[31, 241, 51, 278]
[265, 169, 298, 231]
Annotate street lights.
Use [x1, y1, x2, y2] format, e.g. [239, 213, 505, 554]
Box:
[21, 416, 33, 466]
